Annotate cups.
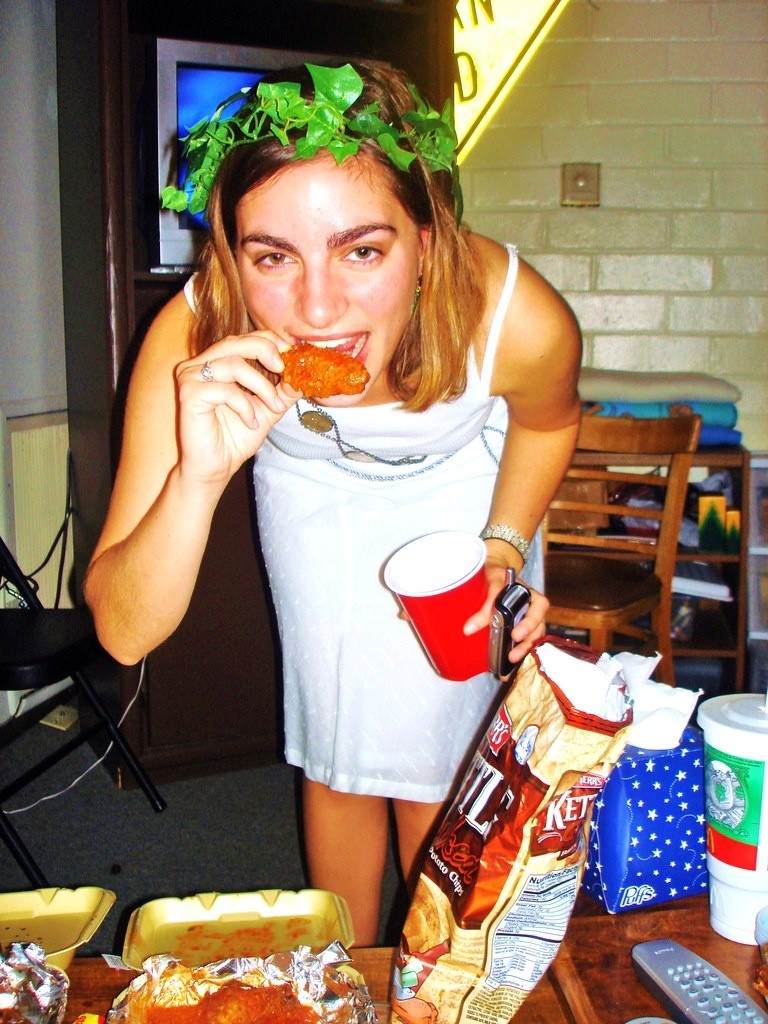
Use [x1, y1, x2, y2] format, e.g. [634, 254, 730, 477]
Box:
[384, 531, 496, 680]
[696, 692, 768, 944]
[755, 906, 768, 965]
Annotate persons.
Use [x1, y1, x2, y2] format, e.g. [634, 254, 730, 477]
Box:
[82, 57, 582, 948]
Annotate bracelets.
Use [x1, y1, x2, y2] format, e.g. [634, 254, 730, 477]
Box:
[479, 524, 531, 564]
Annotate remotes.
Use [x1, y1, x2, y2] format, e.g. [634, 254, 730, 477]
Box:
[631, 939, 768, 1024]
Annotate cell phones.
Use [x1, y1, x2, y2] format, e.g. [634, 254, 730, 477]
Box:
[487, 567, 532, 682]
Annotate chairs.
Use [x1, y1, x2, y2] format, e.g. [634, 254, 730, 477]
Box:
[0, 536, 168, 888]
[541, 414, 700, 687]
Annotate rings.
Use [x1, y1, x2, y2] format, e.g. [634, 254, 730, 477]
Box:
[200, 359, 213, 382]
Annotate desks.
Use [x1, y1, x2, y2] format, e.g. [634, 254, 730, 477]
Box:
[64, 891, 768, 1024]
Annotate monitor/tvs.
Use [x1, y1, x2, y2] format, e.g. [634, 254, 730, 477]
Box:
[138, 37, 390, 275]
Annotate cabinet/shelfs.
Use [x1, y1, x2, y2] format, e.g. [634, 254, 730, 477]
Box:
[55, 0, 456, 789]
[544, 445, 767, 692]
